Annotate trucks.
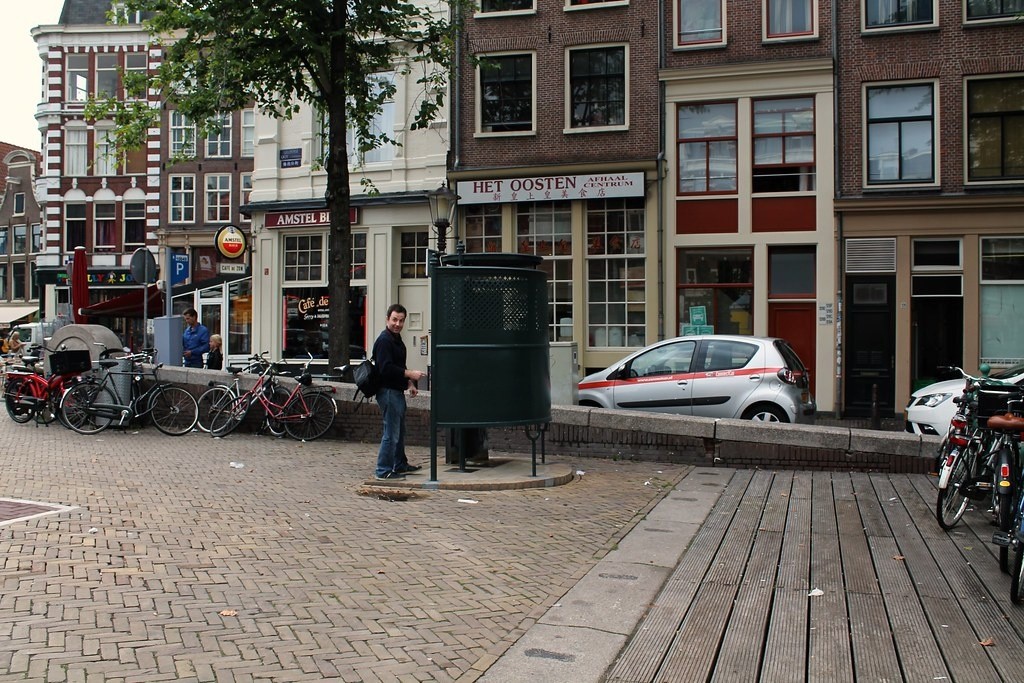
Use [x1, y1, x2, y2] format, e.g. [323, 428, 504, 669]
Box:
[6, 322, 54, 356]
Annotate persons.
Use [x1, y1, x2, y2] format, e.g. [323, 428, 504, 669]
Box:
[0, 331, 9, 355]
[183, 308, 210, 368]
[9, 331, 26, 353]
[206, 334, 223, 370]
[371, 304, 426, 481]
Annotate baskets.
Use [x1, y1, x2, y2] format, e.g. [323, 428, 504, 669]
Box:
[978, 385, 1024, 430]
[49, 350, 92, 374]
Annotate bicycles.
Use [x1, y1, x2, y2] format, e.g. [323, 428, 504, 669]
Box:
[934, 364, 1024, 608]
[1, 343, 350, 442]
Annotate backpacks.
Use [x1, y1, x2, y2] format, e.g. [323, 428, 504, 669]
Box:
[0, 339, 9, 353]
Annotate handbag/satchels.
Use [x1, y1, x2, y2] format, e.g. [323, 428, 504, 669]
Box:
[353, 360, 380, 399]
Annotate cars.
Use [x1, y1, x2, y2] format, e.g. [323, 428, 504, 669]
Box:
[578, 334, 816, 424]
[902, 366, 1024, 438]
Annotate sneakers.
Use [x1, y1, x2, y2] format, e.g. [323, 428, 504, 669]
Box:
[393, 463, 422, 474]
[375, 471, 406, 480]
